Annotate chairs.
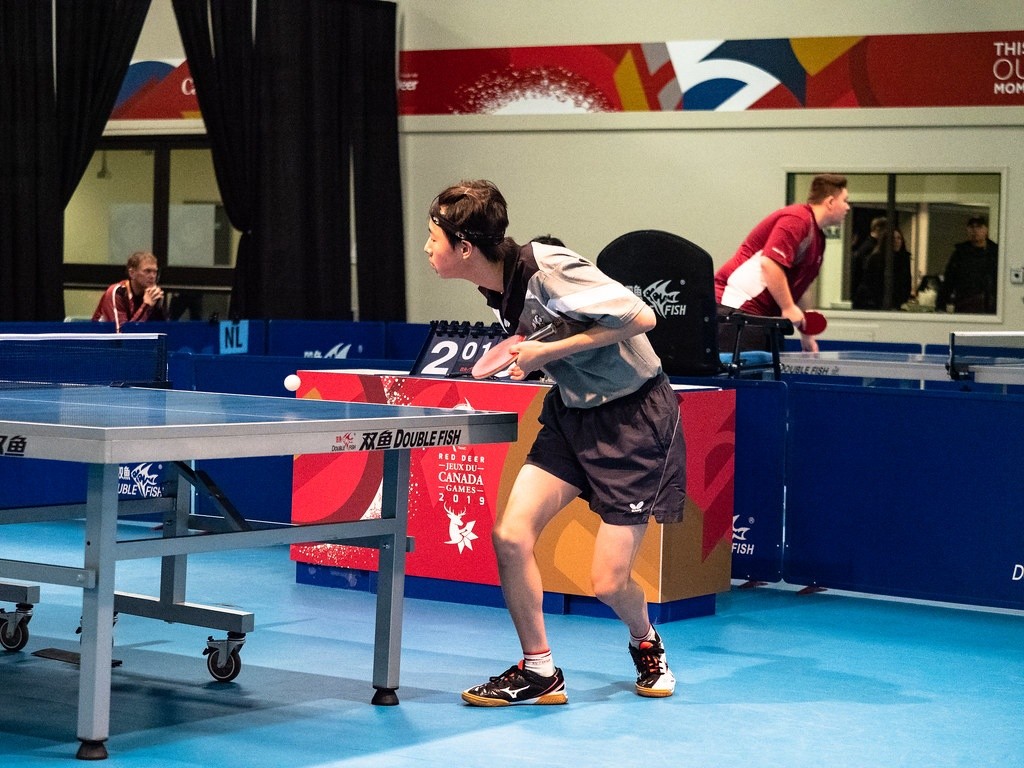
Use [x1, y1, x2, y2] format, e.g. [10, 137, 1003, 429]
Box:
[597, 229, 794, 383]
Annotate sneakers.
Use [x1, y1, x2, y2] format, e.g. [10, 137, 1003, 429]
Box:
[628, 623, 676, 697]
[461, 660, 569, 705]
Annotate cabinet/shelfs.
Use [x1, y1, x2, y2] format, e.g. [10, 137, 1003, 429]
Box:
[107, 200, 233, 271]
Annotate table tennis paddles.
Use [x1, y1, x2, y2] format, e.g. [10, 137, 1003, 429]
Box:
[796, 310, 829, 337]
[470, 322, 558, 378]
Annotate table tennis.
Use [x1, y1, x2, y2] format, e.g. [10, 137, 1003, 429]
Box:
[285, 374, 301, 392]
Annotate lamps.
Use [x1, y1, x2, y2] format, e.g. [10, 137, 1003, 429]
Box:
[96, 151, 113, 182]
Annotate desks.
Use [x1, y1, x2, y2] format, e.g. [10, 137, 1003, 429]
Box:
[1, 381, 519, 761]
[291, 368, 736, 625]
[776, 350, 1024, 386]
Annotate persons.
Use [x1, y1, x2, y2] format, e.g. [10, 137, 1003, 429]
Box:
[847, 217, 914, 312]
[91, 251, 169, 333]
[713, 173, 852, 355]
[422, 179, 684, 706]
[936, 212, 999, 317]
